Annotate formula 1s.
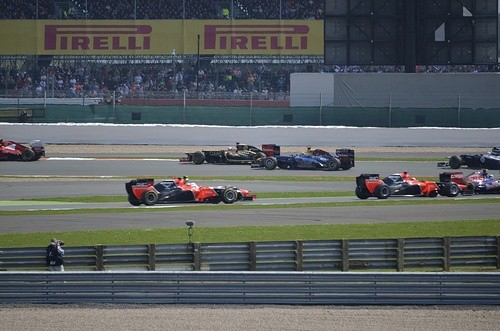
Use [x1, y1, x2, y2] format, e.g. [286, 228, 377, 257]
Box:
[355, 172, 440, 199]
[179, 144, 355, 171]
[448, 147, 500, 170]
[0, 138, 45, 162]
[125, 175, 257, 206]
[436, 169, 500, 198]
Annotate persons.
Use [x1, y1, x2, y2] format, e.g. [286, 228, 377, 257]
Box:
[177, 175, 188, 184]
[402, 171, 408, 180]
[18, 110, 30, 122]
[222, 7, 229, 19]
[243, 0, 325, 19]
[333, 64, 362, 72]
[307, 147, 313, 153]
[0, 138, 11, 146]
[46, 238, 65, 271]
[0, 0, 75, 19]
[83, 0, 223, 19]
[307, 62, 313, 72]
[0, 61, 288, 100]
[425, 64, 500, 73]
[480, 170, 487, 177]
